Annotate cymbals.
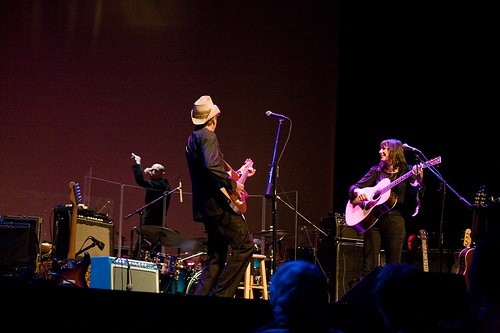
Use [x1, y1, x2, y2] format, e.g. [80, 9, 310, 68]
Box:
[135, 226, 185, 247]
[180, 235, 224, 255]
[253, 229, 292, 238]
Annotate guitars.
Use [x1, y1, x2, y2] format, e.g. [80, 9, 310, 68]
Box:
[456, 191, 486, 289]
[225, 157, 253, 215]
[345, 156, 442, 234]
[418, 229, 430, 273]
[48, 182, 91, 289]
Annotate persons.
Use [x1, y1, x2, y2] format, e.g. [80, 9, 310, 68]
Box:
[348, 139, 423, 279]
[258, 234, 500, 333]
[186, 96, 256, 297]
[131, 153, 172, 261]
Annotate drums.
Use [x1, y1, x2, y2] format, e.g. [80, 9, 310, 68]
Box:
[116, 246, 205, 294]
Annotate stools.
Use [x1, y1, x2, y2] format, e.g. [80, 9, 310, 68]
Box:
[234, 254, 269, 300]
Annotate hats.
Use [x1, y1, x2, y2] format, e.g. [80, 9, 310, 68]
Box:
[190, 95, 221, 125]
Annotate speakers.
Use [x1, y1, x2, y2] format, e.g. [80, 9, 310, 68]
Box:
[0, 214, 42, 276]
[90, 256, 159, 293]
[337, 266, 470, 323]
[319, 241, 369, 304]
[53, 209, 114, 260]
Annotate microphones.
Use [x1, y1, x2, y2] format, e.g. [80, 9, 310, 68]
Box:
[179, 177, 183, 202]
[92, 236, 105, 250]
[402, 144, 419, 152]
[264, 111, 290, 121]
[126, 266, 133, 290]
